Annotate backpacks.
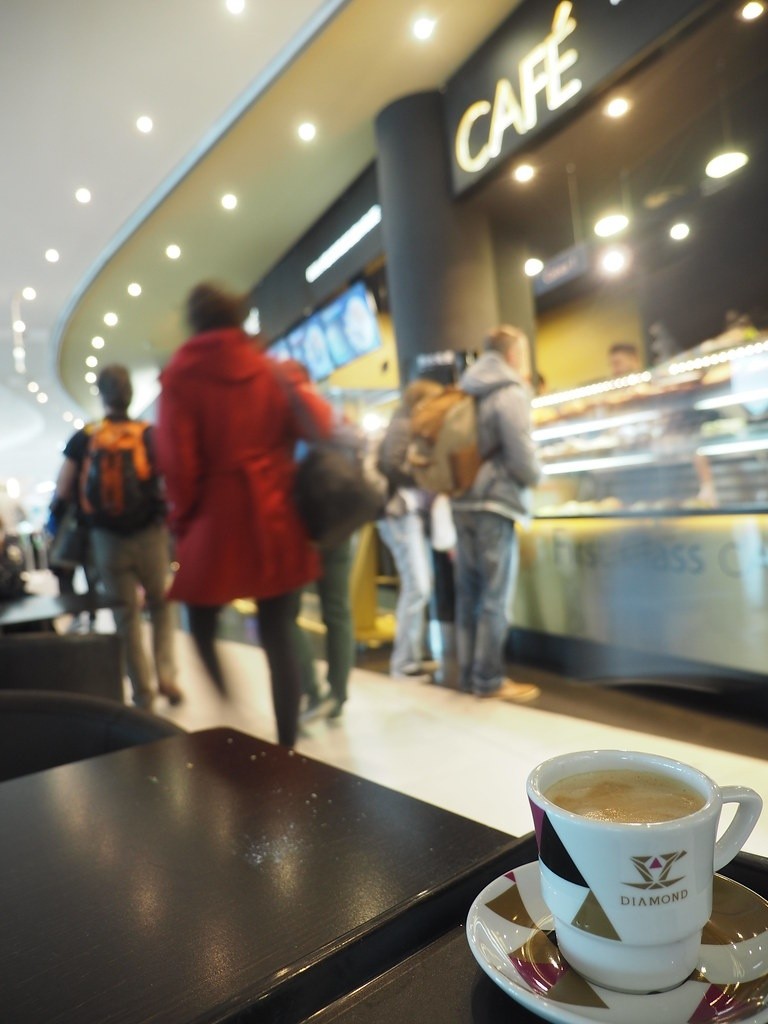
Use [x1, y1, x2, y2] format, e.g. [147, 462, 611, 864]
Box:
[408, 383, 485, 499]
[77, 420, 159, 535]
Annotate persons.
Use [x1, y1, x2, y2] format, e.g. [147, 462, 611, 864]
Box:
[157, 283, 338, 759]
[0, 275, 757, 723]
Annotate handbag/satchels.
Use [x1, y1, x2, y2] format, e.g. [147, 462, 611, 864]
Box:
[291, 389, 385, 552]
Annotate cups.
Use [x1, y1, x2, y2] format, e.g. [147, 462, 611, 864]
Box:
[526, 750, 763, 996]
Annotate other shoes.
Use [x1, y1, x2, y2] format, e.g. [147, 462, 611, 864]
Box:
[474, 680, 541, 703]
[324, 709, 343, 728]
[66, 618, 82, 635]
[297, 682, 336, 722]
[356, 641, 394, 663]
[390, 663, 431, 684]
[158, 682, 183, 706]
[89, 620, 96, 634]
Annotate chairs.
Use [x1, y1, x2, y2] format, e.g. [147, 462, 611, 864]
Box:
[0, 633, 189, 783]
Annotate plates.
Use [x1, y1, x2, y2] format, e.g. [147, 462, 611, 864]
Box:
[466, 860, 768, 1024]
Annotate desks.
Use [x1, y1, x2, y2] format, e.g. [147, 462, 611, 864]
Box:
[0, 594, 127, 626]
[0, 726, 539, 1024]
